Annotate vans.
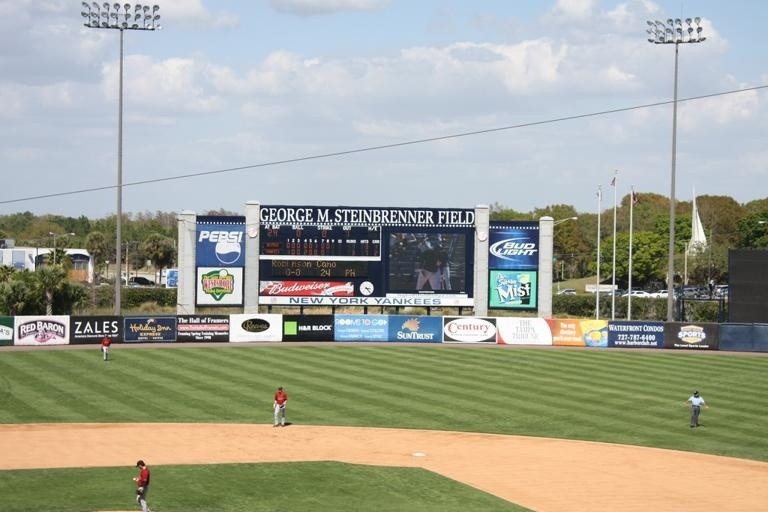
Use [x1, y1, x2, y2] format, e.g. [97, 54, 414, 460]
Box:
[555, 288, 577, 295]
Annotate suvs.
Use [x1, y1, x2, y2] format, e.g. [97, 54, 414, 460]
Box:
[128, 276, 156, 288]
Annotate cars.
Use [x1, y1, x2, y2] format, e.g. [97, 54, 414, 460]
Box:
[608, 284, 729, 300]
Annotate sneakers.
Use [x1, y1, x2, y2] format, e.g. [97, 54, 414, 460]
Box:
[273, 424, 285, 427]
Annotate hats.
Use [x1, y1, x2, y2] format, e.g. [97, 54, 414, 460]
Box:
[135, 460, 144, 468]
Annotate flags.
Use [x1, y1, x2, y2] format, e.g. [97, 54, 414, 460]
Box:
[634, 191, 640, 206]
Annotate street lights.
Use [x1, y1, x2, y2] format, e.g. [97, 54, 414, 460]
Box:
[104, 260, 110, 280]
[80, 0, 161, 319]
[646, 16, 707, 323]
[48, 230, 76, 267]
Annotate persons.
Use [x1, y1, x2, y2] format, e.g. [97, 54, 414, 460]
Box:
[100, 333, 111, 361]
[133, 460, 151, 512]
[272, 385, 288, 427]
[684, 390, 709, 427]
[389, 232, 453, 290]
